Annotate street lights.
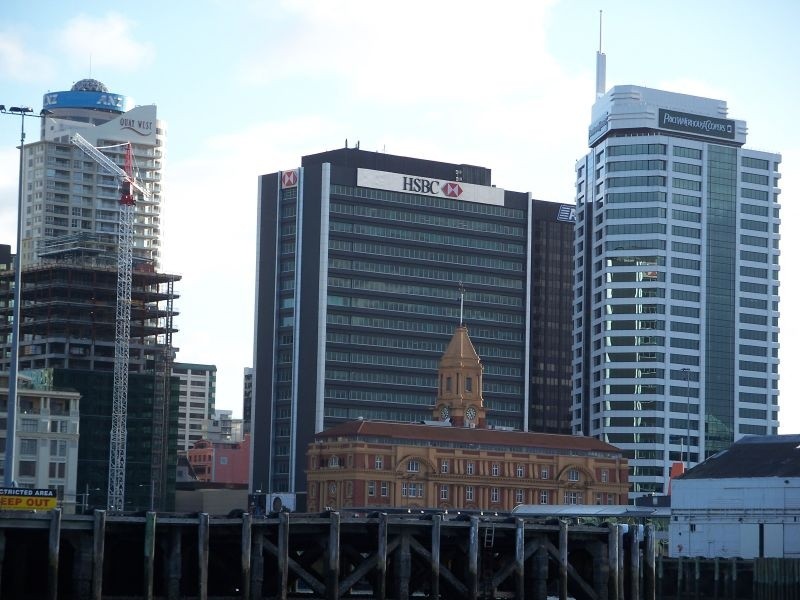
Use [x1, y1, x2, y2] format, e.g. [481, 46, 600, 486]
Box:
[0, 103, 56, 472]
[679, 367, 690, 472]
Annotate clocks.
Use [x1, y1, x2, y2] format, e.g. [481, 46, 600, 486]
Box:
[441, 407, 448, 419]
[466, 407, 477, 420]
